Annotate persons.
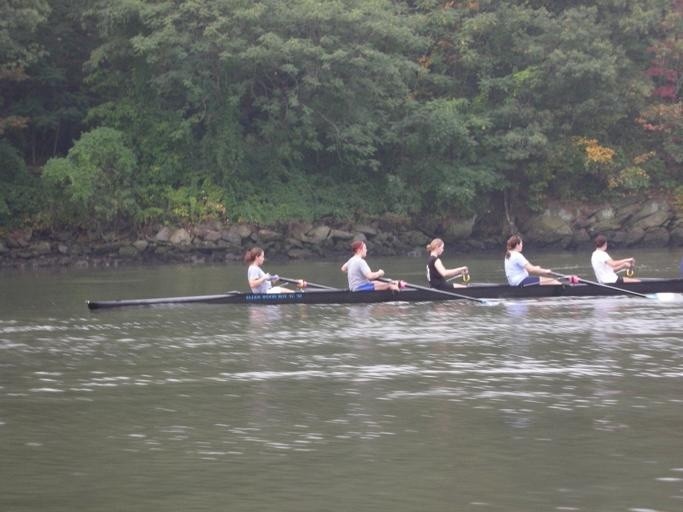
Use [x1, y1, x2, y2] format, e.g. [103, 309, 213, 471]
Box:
[589, 236, 642, 283]
[423, 238, 468, 288]
[339, 241, 402, 291]
[246, 247, 295, 294]
[503, 235, 562, 286]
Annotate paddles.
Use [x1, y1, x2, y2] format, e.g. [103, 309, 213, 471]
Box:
[547, 272, 683, 304]
[375, 278, 515, 306]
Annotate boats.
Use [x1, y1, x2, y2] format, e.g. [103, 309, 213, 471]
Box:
[85, 278, 683, 308]
